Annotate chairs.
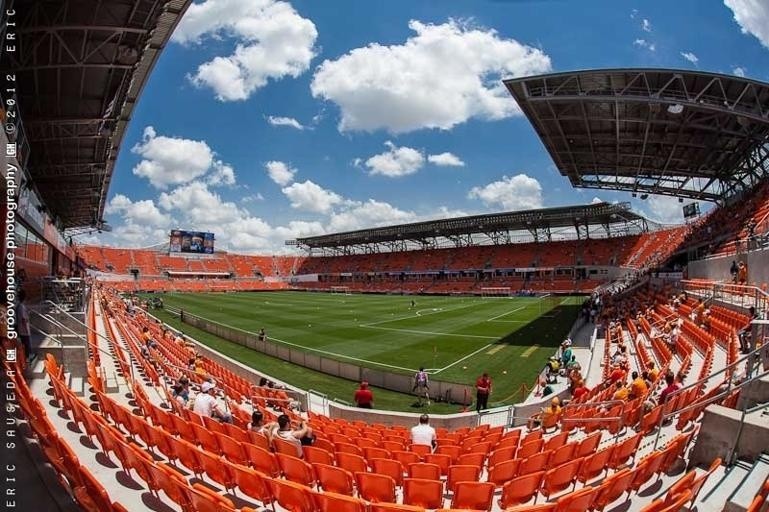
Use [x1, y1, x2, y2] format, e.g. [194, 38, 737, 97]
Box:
[1, 180, 769, 512]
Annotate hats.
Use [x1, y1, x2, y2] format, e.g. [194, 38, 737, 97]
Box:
[202, 382, 215, 393]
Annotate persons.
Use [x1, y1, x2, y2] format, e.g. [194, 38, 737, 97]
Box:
[1, 172, 769, 457]
